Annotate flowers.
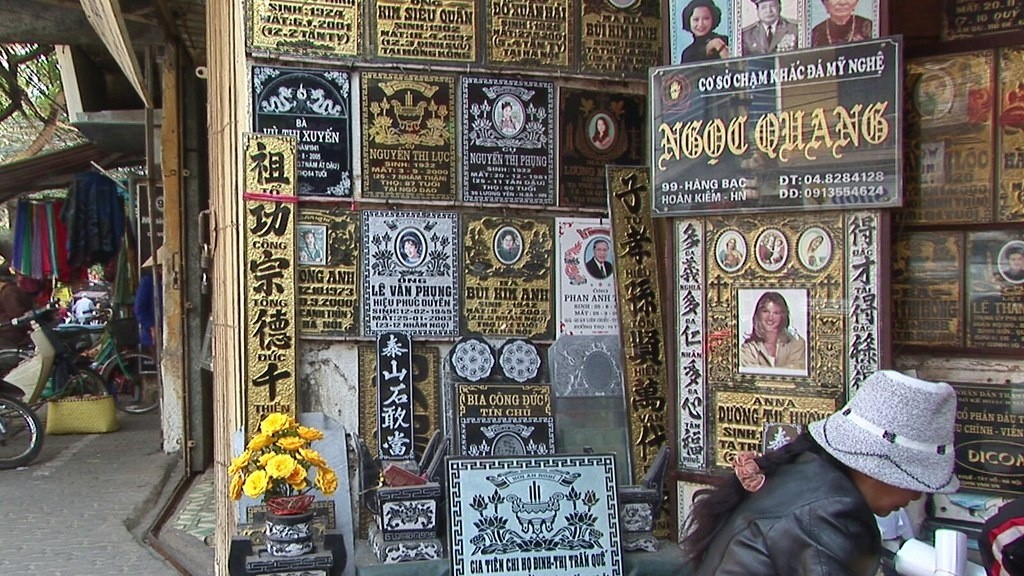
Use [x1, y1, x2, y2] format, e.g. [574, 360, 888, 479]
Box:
[226, 413, 339, 501]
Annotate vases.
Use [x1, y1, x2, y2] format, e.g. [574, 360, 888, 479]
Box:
[369, 483, 443, 566]
[266, 510, 313, 558]
[616, 484, 660, 552]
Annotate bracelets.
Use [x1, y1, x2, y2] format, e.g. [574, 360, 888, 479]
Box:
[717, 44, 727, 54]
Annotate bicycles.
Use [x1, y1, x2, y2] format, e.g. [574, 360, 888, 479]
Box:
[0, 308, 160, 416]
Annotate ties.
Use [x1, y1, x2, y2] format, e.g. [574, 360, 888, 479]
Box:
[767, 26, 772, 46]
[600, 265, 604, 278]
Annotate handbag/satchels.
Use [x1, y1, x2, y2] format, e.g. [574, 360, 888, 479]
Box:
[47, 378, 120, 433]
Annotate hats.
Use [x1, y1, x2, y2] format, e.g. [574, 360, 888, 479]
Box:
[807, 370, 961, 492]
[141, 245, 164, 268]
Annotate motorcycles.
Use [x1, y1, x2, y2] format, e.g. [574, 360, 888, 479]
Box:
[0, 297, 110, 470]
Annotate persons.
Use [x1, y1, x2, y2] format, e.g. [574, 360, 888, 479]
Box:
[812, 0, 872, 48]
[591, 117, 614, 150]
[741, 292, 806, 369]
[742, 0, 798, 55]
[587, 240, 613, 279]
[1003, 247, 1024, 280]
[802, 232, 824, 267]
[497, 232, 520, 261]
[401, 233, 421, 264]
[681, 369, 960, 576]
[759, 233, 784, 264]
[0, 266, 35, 349]
[679, 0, 730, 63]
[718, 235, 743, 268]
[135, 245, 164, 362]
[298, 230, 324, 263]
[499, 102, 520, 133]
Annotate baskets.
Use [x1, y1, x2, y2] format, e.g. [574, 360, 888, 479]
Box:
[109, 318, 139, 347]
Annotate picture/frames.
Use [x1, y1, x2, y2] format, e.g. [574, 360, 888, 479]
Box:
[667, 465, 735, 544]
[644, 33, 905, 210]
[667, 205, 892, 475]
[886, 31, 1024, 357]
